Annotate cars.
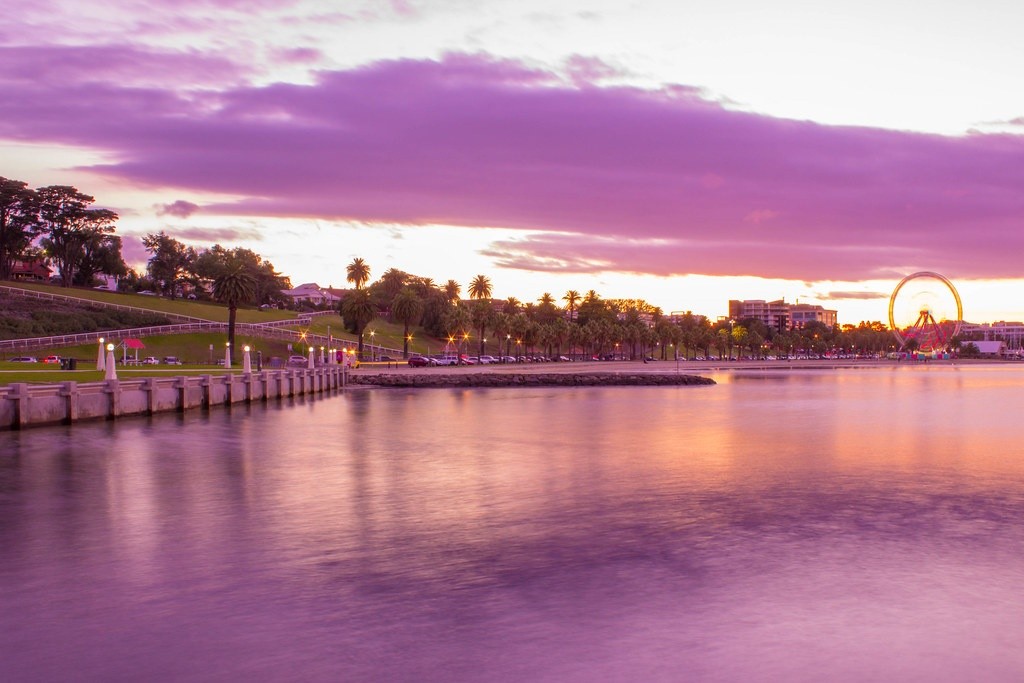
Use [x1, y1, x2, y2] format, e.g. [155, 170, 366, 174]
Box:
[429, 358, 444, 366]
[592, 355, 658, 361]
[44, 355, 61, 364]
[362, 356, 396, 362]
[678, 354, 881, 361]
[94, 285, 110, 290]
[10, 357, 37, 362]
[462, 355, 573, 365]
[137, 290, 156, 295]
[120, 355, 135, 362]
[162, 356, 182, 365]
[143, 357, 159, 365]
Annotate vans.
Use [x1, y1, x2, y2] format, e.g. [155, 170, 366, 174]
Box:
[289, 356, 308, 365]
[439, 356, 458, 365]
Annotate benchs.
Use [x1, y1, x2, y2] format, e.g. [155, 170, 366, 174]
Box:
[129, 362, 143, 366]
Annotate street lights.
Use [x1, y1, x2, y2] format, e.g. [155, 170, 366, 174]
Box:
[371, 332, 374, 362]
[302, 334, 305, 356]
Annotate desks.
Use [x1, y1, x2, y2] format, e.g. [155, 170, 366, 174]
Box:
[126, 360, 140, 366]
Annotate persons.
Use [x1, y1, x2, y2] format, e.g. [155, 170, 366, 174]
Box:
[388, 361, 444, 369]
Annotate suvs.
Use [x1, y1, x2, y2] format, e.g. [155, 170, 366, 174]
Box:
[408, 356, 436, 368]
